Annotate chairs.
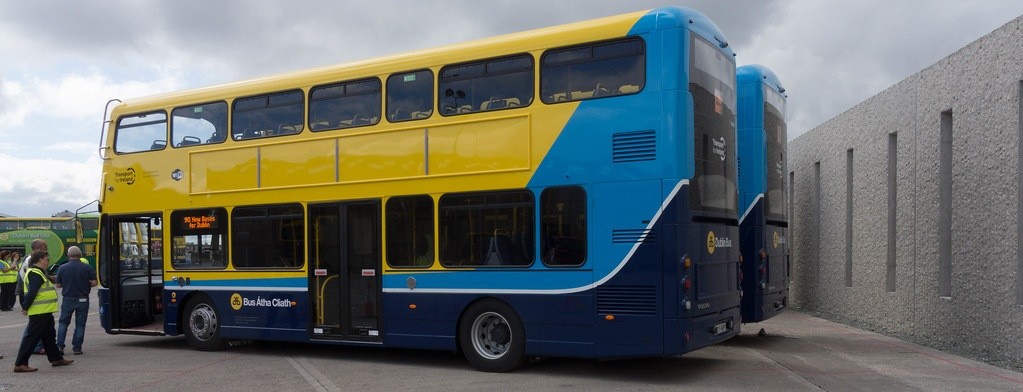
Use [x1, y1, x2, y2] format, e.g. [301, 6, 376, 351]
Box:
[485, 228, 516, 265]
[149, 82, 622, 151]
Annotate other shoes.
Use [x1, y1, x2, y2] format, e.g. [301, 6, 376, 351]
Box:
[35, 348, 47, 355]
[51, 358, 74, 367]
[74, 350, 83, 355]
[58, 347, 65, 355]
[13, 365, 38, 373]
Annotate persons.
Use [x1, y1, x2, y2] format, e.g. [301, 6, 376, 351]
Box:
[0, 251, 20, 311]
[14, 239, 99, 373]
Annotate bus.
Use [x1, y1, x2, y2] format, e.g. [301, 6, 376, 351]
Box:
[75, 5, 744, 373]
[264, 64, 793, 324]
[0, 210, 187, 275]
